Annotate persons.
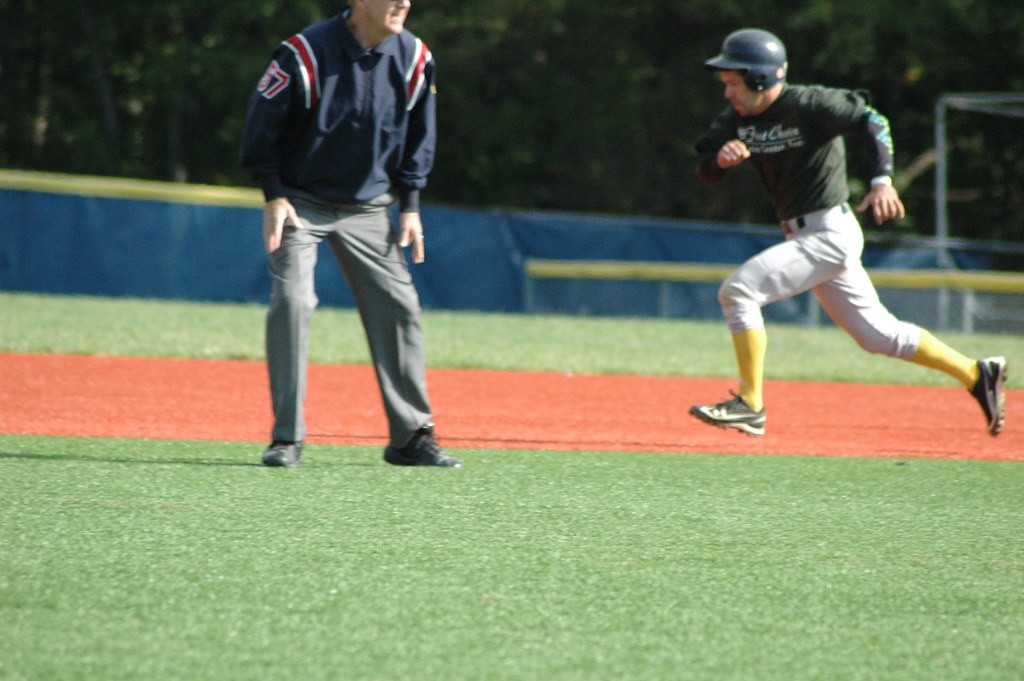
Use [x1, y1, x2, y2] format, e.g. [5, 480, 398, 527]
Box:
[239, 0, 465, 468]
[690, 28, 1009, 436]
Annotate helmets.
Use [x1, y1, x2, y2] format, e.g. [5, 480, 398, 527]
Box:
[704, 28, 788, 93]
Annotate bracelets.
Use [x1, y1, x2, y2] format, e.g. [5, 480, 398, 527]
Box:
[870, 175, 892, 187]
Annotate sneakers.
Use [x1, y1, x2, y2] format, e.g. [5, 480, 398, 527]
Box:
[263, 440, 303, 467]
[382, 428, 460, 468]
[966, 356, 1008, 436]
[689, 390, 766, 437]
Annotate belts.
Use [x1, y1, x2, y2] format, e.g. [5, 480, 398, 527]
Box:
[781, 202, 848, 235]
